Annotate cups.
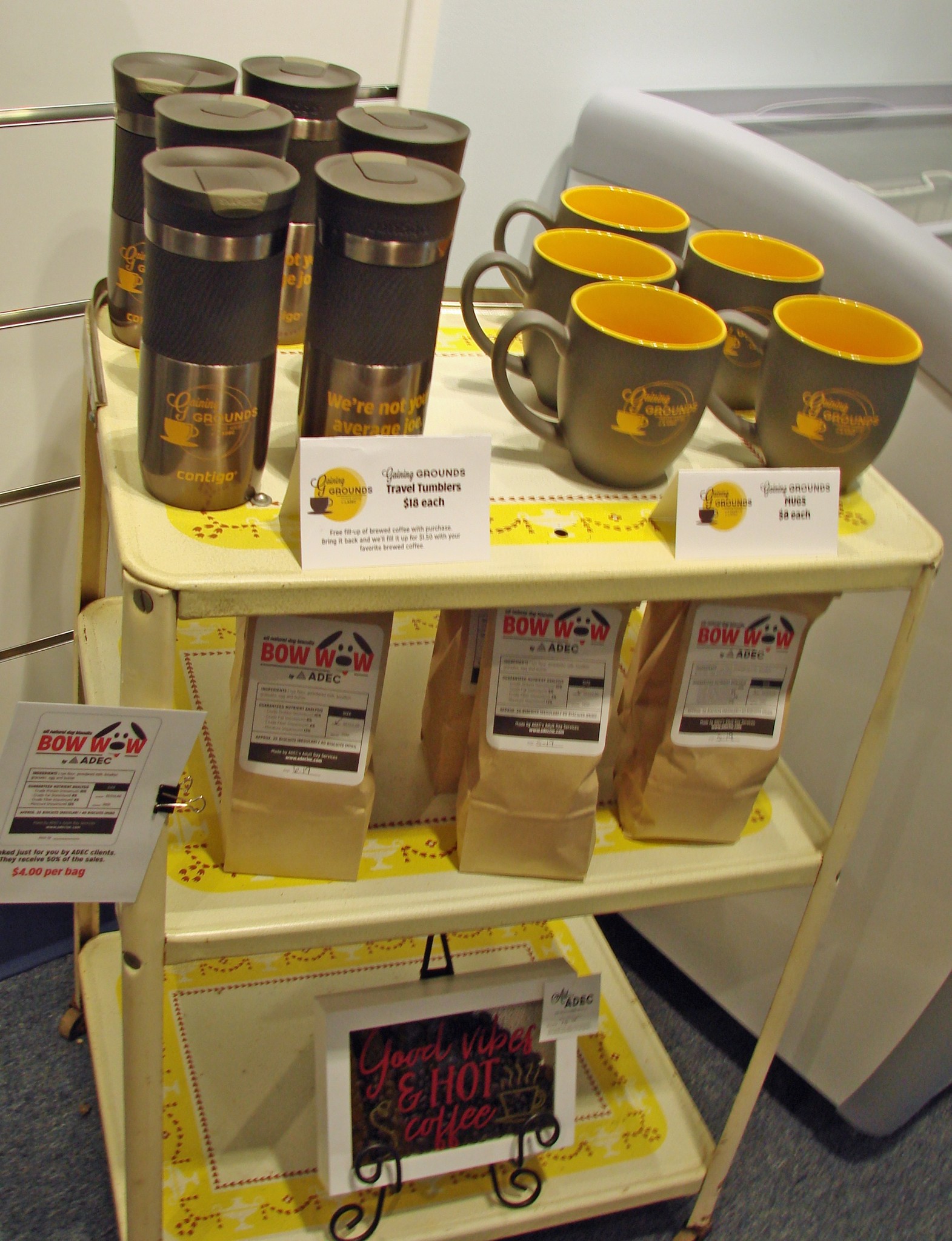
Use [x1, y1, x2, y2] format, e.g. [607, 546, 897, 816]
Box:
[460, 181, 924, 502]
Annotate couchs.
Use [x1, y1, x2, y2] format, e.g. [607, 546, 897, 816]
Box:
[511, 87, 952, 1147]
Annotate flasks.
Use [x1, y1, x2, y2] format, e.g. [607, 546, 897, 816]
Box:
[101, 44, 464, 510]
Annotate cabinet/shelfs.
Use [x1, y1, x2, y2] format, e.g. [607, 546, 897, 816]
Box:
[54, 264, 948, 1241]
